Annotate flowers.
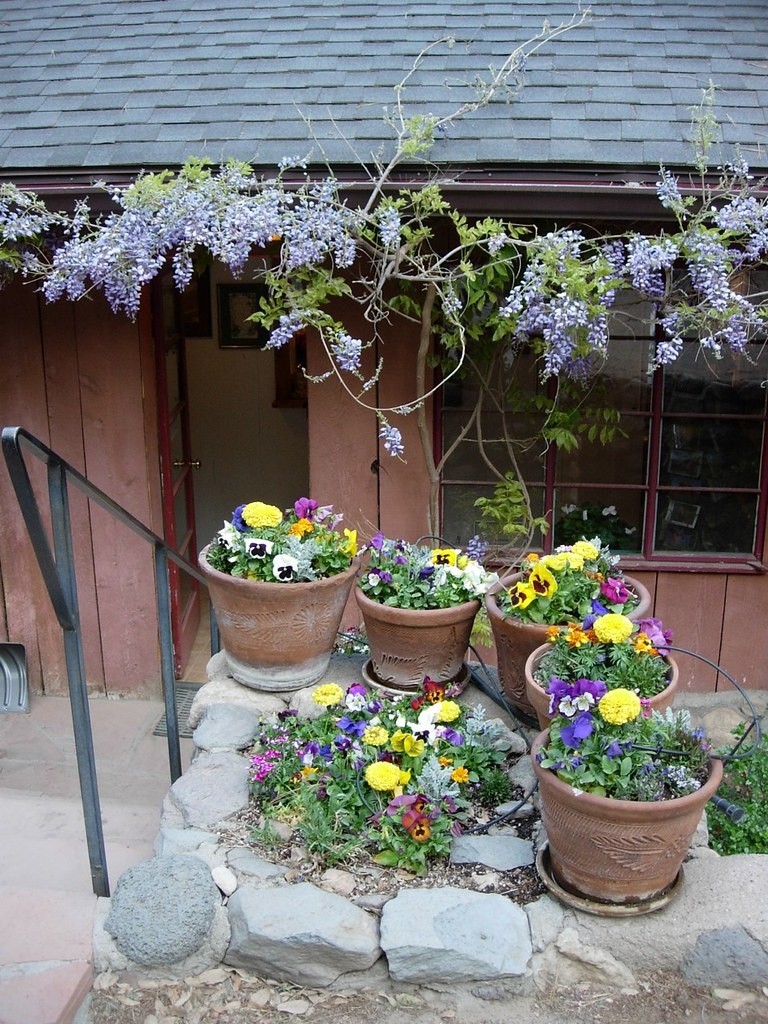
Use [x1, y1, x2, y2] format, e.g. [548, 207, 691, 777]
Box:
[205, 497, 713, 802]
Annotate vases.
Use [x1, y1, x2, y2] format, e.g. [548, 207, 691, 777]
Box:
[354, 584, 482, 700]
[524, 643, 679, 732]
[485, 571, 651, 720]
[529, 727, 725, 920]
[198, 540, 362, 692]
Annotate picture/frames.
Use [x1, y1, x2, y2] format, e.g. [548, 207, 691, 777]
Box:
[270, 252, 307, 409]
[217, 283, 272, 348]
[173, 258, 212, 337]
